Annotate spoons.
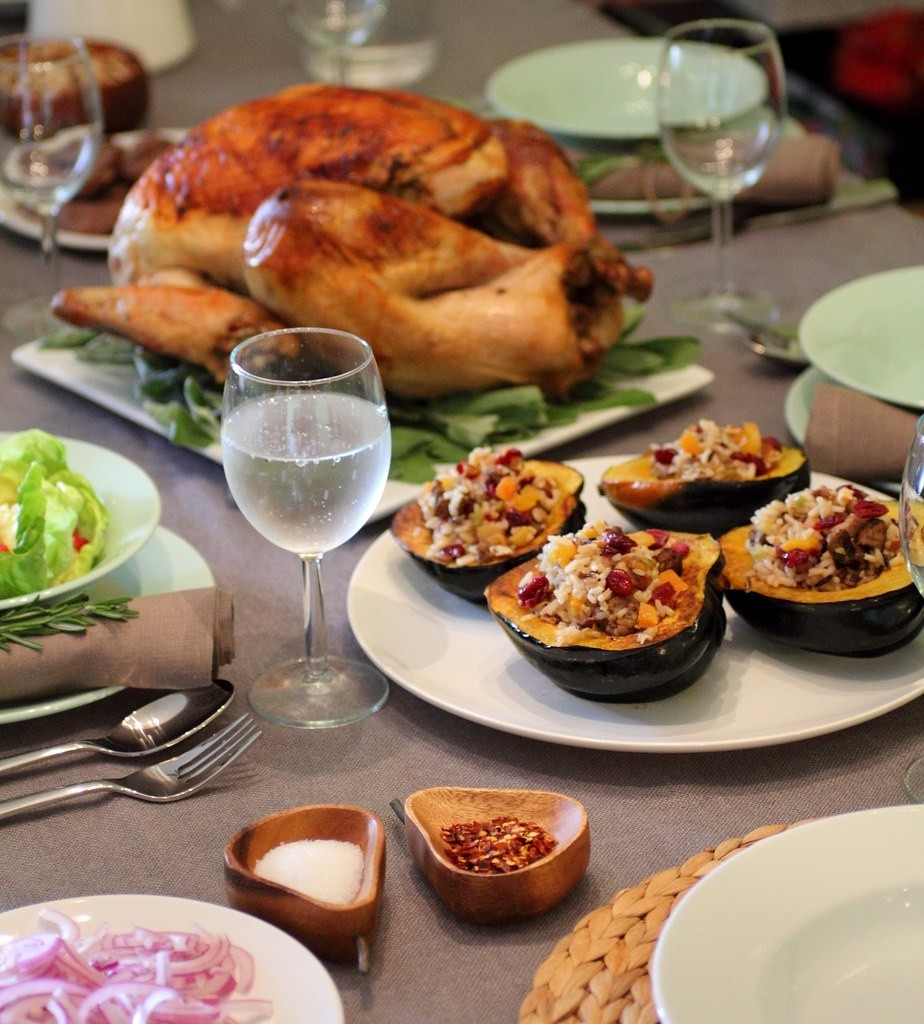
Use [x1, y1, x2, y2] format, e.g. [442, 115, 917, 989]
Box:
[1, 681, 233, 774]
[751, 330, 812, 367]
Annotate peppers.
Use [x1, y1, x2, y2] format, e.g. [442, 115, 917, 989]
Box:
[441, 814, 558, 874]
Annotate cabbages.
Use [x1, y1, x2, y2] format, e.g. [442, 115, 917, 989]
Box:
[0, 426, 109, 601]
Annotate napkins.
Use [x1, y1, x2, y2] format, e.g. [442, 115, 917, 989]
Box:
[550, 135, 841, 209]
[803, 375, 920, 487]
[0, 585, 239, 695]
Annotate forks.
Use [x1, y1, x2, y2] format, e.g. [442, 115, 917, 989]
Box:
[2, 714, 264, 820]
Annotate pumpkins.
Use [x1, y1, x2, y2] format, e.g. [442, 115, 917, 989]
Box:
[390, 418, 923, 704]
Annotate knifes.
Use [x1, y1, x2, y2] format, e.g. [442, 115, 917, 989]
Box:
[608, 178, 899, 252]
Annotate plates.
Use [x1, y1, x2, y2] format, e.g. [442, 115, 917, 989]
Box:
[648, 799, 924, 1022]
[1, 122, 184, 255]
[2, 424, 160, 609]
[349, 450, 924, 753]
[13, 320, 711, 521]
[787, 261, 923, 492]
[485, 39, 784, 217]
[1, 896, 345, 1024]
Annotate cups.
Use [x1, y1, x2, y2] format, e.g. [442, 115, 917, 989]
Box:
[306, 0, 437, 91]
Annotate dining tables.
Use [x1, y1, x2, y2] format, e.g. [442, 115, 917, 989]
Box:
[1, 0, 923, 1024]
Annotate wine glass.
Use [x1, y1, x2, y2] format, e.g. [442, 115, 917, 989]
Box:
[658, 20, 786, 335]
[2, 30, 102, 341]
[219, 328, 390, 730]
[895, 415, 924, 802]
[284, 0, 384, 85]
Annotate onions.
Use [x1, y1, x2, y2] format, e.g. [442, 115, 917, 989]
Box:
[0, 902, 272, 1024]
